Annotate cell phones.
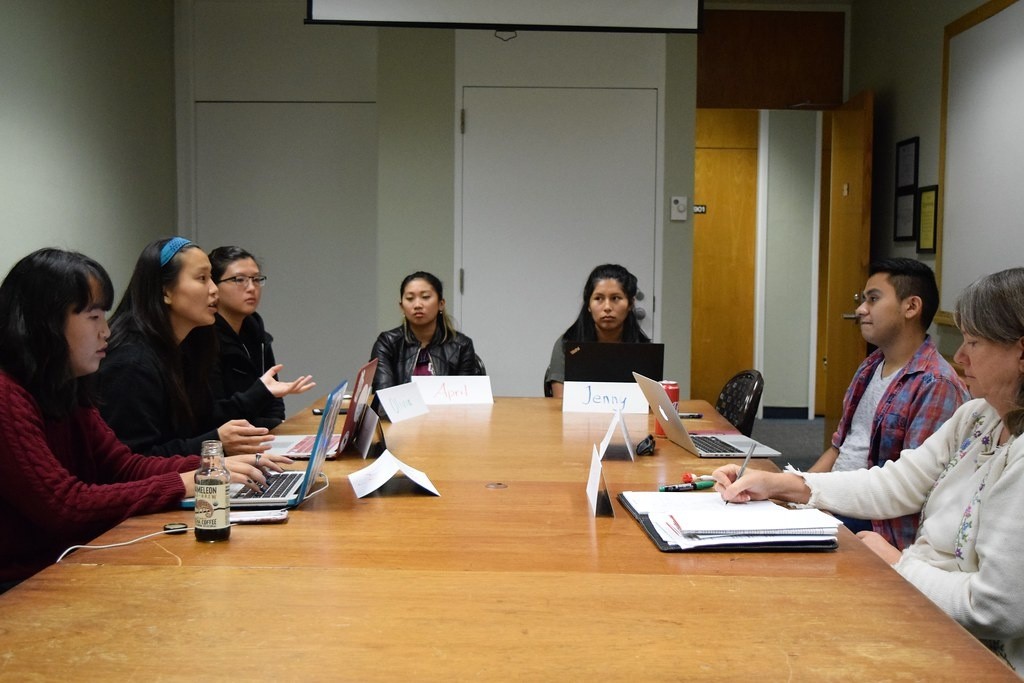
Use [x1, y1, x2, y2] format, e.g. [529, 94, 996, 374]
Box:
[313, 408, 349, 415]
[229, 510, 288, 523]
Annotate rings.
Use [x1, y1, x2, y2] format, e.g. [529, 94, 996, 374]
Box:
[254, 454, 261, 469]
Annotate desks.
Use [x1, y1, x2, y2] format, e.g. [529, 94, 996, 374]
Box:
[0, 395, 1024, 682]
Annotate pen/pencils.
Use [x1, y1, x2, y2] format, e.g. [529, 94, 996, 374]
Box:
[678, 412, 703, 419]
[659, 481, 714, 492]
[725, 442, 757, 505]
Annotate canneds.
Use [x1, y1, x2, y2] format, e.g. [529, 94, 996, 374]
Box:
[654, 380, 679, 438]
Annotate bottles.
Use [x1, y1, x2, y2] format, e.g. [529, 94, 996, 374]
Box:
[193, 440, 231, 542]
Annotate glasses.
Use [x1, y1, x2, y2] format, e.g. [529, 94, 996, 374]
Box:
[217, 274, 267, 288]
[636, 435, 656, 456]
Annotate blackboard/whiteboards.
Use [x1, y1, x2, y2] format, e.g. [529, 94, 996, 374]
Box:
[932, 0, 1024, 325]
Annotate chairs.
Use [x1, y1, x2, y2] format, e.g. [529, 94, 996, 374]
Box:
[715, 369, 764, 438]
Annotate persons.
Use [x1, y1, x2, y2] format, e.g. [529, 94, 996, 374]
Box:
[368, 271, 477, 417]
[712, 268, 1024, 678]
[207, 245, 286, 427]
[0, 247, 294, 593]
[94, 236, 316, 459]
[547, 264, 652, 399]
[804, 258, 971, 551]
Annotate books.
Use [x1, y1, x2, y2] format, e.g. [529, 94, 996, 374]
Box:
[670, 508, 839, 536]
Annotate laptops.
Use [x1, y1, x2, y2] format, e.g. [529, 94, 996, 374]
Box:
[258, 358, 379, 458]
[631, 371, 782, 459]
[180, 381, 349, 509]
[563, 341, 663, 384]
